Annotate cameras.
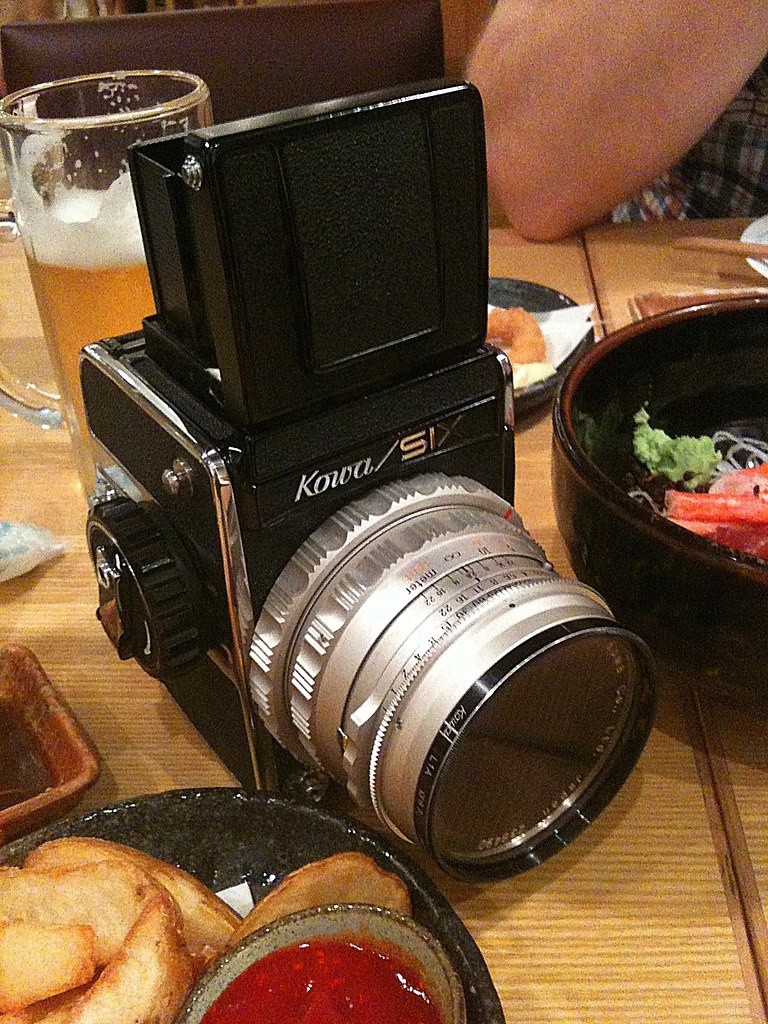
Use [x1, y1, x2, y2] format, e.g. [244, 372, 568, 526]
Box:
[80, 75, 660, 884]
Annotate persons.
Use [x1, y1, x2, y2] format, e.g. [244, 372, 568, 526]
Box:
[471, 0, 768, 243]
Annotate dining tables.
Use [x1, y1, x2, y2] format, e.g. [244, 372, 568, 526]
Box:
[0, 218, 768, 1023]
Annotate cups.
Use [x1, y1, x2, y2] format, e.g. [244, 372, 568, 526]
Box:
[0, 70, 213, 502]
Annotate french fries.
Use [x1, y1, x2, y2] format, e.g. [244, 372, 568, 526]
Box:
[0, 835, 411, 1024]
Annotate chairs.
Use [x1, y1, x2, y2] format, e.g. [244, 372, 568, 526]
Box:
[0, 0, 447, 160]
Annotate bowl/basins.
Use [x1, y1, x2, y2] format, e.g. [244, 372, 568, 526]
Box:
[0, 644, 100, 843]
[553, 289, 768, 694]
[1, 786, 506, 1023]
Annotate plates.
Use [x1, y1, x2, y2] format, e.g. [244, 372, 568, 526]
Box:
[741, 214, 768, 278]
[489, 273, 594, 411]
